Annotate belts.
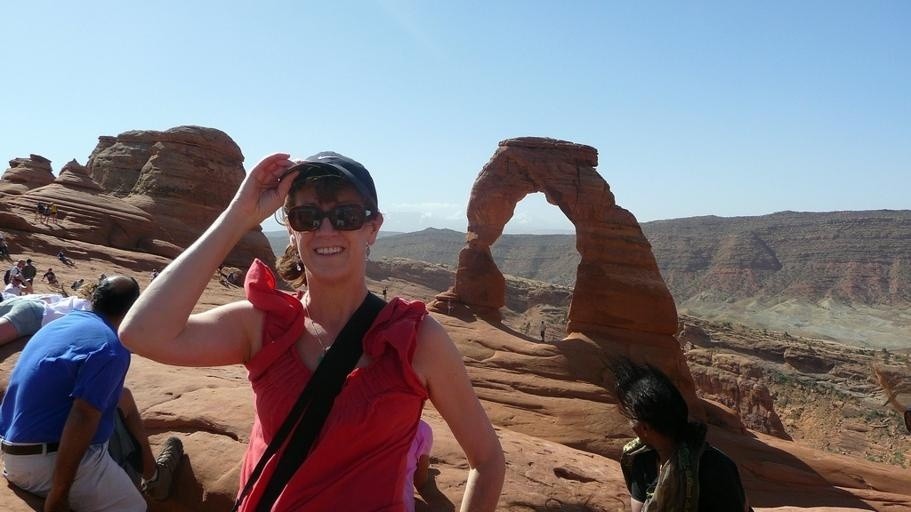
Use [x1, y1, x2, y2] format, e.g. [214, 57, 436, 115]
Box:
[0, 438, 61, 455]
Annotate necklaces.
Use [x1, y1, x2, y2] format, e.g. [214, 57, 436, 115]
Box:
[305, 293, 331, 367]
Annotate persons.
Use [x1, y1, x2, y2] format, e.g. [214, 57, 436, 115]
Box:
[0, 276, 183, 512]
[519, 321, 546, 340]
[615, 363, 753, 512]
[0, 237, 157, 345]
[38, 202, 57, 224]
[118, 151, 504, 512]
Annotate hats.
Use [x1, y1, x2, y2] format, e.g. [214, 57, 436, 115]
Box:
[277, 151, 379, 217]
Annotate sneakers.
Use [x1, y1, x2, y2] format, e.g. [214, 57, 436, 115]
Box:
[139, 437, 184, 503]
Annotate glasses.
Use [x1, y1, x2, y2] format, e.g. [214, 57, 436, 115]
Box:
[286, 202, 376, 231]
